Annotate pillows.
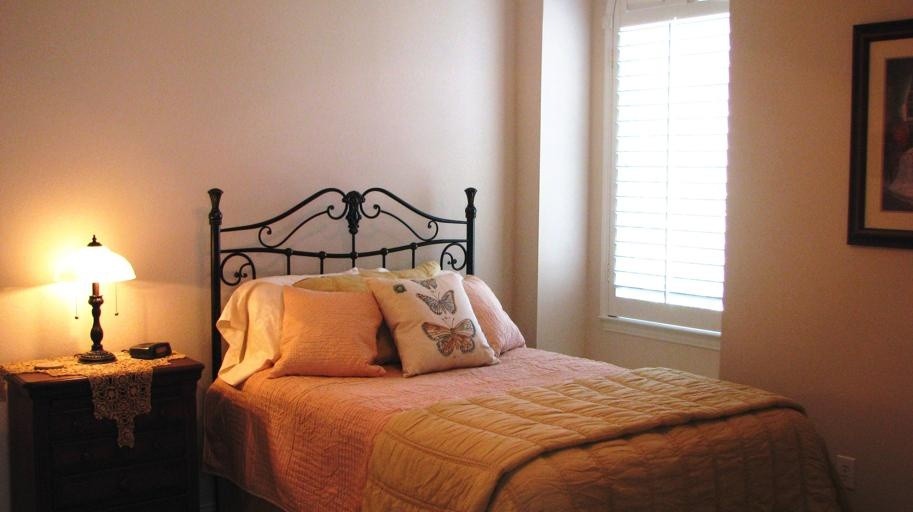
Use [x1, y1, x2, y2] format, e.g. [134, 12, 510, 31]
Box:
[266, 259, 527, 379]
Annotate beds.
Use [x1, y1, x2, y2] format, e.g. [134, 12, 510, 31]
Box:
[197, 188, 851, 511]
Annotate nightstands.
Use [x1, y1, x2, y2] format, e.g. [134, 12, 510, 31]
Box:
[0, 348, 205, 512]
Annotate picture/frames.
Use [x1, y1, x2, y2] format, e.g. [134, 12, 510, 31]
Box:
[848, 15, 912, 250]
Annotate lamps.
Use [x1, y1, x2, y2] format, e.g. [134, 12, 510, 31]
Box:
[54, 234, 136, 364]
